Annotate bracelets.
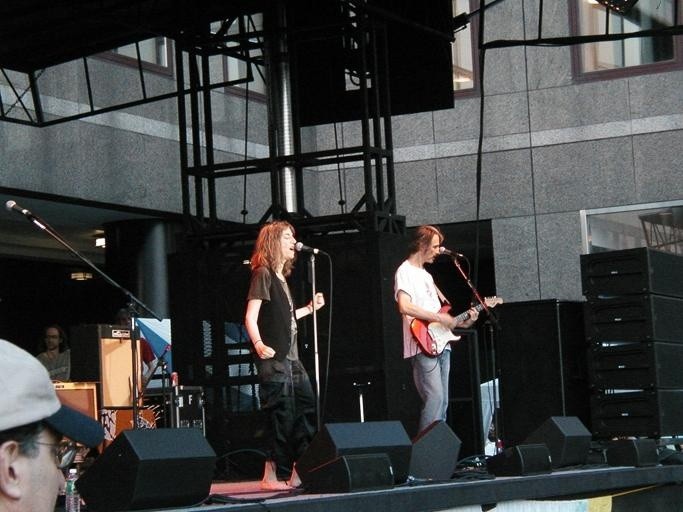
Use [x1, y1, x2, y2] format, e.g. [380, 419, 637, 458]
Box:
[253, 339, 262, 348]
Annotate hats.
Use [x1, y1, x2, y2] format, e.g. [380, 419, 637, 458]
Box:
[0, 339, 106, 447]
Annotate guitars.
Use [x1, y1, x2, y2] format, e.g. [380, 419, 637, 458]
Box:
[411, 294, 504, 358]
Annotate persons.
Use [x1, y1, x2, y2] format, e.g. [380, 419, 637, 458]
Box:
[0, 339, 104, 512]
[244, 221, 325, 492]
[392, 224, 482, 440]
[116, 310, 156, 388]
[35, 324, 71, 380]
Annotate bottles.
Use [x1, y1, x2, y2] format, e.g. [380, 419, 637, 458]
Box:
[64, 468, 82, 512]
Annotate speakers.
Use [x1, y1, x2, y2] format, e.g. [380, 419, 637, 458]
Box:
[520, 415, 593, 467]
[293, 420, 413, 493]
[68, 322, 144, 409]
[578, 245, 683, 437]
[601, 438, 660, 467]
[409, 419, 463, 483]
[54, 382, 98, 466]
[484, 442, 553, 476]
[98, 408, 158, 456]
[74, 428, 218, 512]
[497, 299, 593, 448]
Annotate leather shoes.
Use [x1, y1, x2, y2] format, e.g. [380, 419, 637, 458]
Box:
[258, 480, 293, 492]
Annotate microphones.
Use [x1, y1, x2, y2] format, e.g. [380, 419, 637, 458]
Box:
[295, 241, 327, 256]
[5, 200, 38, 219]
[439, 246, 464, 258]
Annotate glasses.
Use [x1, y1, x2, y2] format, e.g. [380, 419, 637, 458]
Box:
[25, 437, 63, 467]
